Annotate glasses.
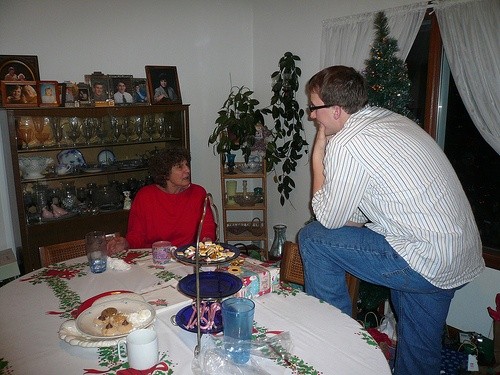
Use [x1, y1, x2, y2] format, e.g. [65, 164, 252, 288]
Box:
[309, 104, 337, 112]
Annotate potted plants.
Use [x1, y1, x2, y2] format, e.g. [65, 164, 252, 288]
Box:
[210, 83, 271, 173]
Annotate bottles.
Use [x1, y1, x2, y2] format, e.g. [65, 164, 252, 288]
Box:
[269, 224, 287, 259]
[24, 176, 153, 220]
[253, 187, 263, 203]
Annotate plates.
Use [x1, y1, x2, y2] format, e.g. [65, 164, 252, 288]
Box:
[178, 271, 243, 300]
[175, 302, 238, 333]
[58, 288, 157, 348]
[55, 149, 170, 173]
[174, 242, 240, 264]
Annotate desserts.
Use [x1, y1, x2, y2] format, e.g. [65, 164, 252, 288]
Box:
[96, 307, 151, 335]
[176, 241, 235, 260]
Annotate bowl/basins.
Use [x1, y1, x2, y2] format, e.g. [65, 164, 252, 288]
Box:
[231, 195, 259, 207]
[240, 162, 260, 172]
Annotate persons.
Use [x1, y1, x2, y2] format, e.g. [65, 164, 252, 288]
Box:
[135, 84, 147, 102]
[41, 88, 56, 103]
[5, 67, 25, 81]
[154, 75, 175, 101]
[7, 86, 28, 103]
[93, 82, 108, 101]
[298, 65, 486, 375]
[107, 150, 218, 257]
[114, 79, 132, 103]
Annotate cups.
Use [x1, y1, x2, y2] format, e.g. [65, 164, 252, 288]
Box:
[118, 328, 158, 371]
[54, 164, 71, 175]
[226, 181, 237, 205]
[84, 231, 107, 273]
[221, 297, 255, 364]
[152, 240, 171, 266]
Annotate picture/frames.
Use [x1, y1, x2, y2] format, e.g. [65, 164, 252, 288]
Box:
[0, 55, 183, 106]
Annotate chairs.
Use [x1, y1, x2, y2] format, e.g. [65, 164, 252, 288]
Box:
[280, 241, 359, 315]
[38, 232, 121, 268]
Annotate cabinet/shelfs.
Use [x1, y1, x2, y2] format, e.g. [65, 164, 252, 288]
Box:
[219, 140, 269, 262]
[4, 104, 192, 273]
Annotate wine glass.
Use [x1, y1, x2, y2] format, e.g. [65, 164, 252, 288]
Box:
[15, 115, 176, 150]
[18, 157, 48, 179]
[225, 154, 237, 175]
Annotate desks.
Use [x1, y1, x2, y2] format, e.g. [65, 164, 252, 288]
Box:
[0, 247, 391, 375]
